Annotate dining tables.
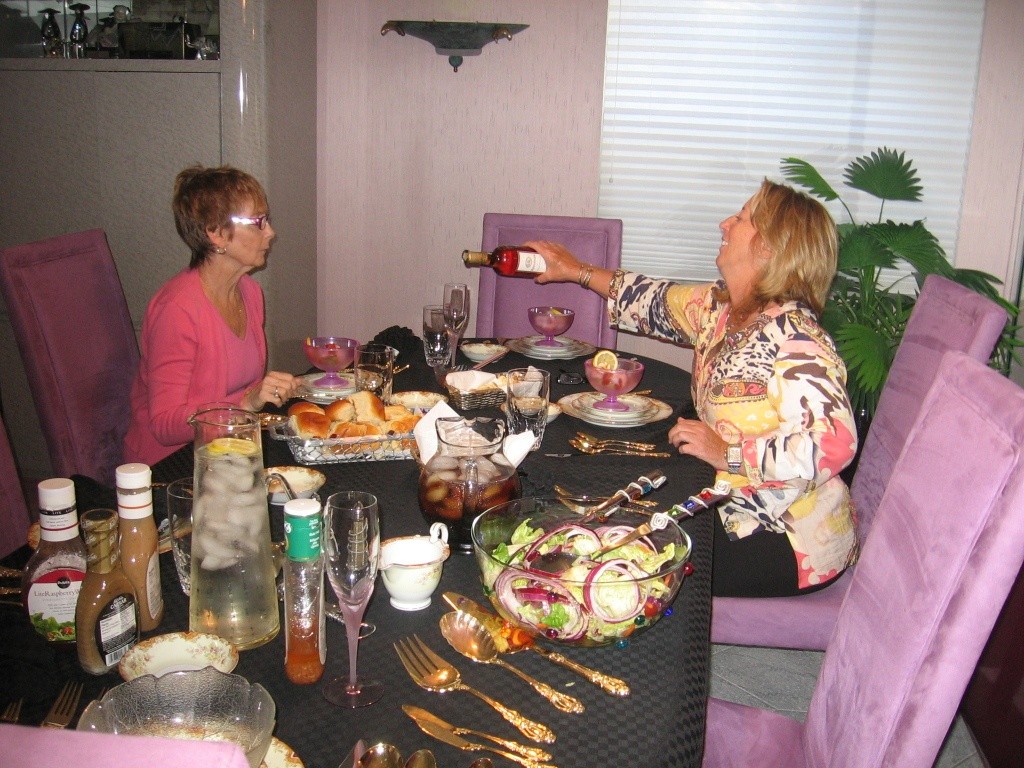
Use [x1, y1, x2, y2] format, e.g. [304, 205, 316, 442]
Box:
[1, 336, 719, 768]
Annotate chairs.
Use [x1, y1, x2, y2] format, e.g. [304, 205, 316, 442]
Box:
[477, 211, 625, 352]
[1, 228, 143, 490]
[701, 349, 1023, 768]
[709, 273, 1010, 655]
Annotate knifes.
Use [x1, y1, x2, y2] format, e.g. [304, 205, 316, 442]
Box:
[442, 587, 628, 699]
[401, 702, 553, 760]
[415, 715, 560, 768]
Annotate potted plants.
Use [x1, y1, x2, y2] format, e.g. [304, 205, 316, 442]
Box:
[775, 141, 1024, 488]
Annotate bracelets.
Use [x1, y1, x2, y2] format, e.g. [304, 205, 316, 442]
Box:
[578, 264, 595, 289]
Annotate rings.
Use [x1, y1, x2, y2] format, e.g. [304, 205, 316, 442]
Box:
[274, 386, 280, 398]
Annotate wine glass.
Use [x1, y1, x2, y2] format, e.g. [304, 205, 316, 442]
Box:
[613, 365, 614, 368]
[318, 489, 386, 705]
[302, 337, 358, 388]
[583, 356, 643, 410]
[527, 307, 576, 347]
[443, 283, 469, 369]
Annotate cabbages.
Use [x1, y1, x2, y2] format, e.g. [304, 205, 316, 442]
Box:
[484, 517, 677, 642]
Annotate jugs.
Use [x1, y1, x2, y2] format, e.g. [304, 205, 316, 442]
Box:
[417, 413, 525, 557]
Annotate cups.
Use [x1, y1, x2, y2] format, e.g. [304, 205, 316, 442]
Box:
[508, 368, 551, 451]
[421, 301, 452, 366]
[165, 406, 300, 651]
[352, 344, 392, 403]
[377, 523, 454, 613]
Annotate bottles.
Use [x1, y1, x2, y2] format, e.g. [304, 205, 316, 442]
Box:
[463, 245, 548, 280]
[28, 464, 164, 676]
[283, 495, 326, 685]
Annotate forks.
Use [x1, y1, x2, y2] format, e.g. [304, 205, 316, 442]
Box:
[2, 674, 88, 729]
[553, 484, 657, 516]
[392, 632, 557, 746]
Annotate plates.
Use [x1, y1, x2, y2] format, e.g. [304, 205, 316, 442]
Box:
[393, 391, 448, 413]
[126, 719, 305, 768]
[563, 388, 672, 428]
[288, 369, 374, 406]
[508, 334, 595, 361]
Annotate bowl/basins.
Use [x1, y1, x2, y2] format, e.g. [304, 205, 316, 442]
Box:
[262, 463, 326, 505]
[500, 398, 561, 426]
[73, 629, 278, 768]
[467, 496, 692, 650]
[458, 338, 508, 364]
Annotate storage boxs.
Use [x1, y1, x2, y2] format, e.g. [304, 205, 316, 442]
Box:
[116, 21, 201, 60]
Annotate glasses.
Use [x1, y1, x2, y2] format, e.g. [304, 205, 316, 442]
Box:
[231, 214, 268, 230]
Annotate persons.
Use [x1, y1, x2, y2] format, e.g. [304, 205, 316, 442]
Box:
[522, 176, 860, 598]
[121, 165, 306, 486]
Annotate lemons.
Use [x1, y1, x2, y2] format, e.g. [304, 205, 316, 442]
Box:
[208, 437, 259, 456]
[593, 349, 618, 372]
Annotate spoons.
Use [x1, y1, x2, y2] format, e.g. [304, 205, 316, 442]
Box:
[569, 431, 672, 458]
[359, 742, 403, 768]
[405, 749, 437, 767]
[439, 614, 585, 716]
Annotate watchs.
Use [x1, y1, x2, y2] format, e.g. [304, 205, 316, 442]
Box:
[724, 444, 744, 474]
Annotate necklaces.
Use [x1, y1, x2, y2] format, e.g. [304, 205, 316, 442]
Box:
[725, 304, 745, 331]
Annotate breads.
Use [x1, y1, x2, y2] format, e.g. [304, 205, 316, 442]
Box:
[287, 391, 422, 454]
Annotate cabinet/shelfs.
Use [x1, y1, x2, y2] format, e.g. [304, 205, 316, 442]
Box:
[1, 57, 223, 330]
